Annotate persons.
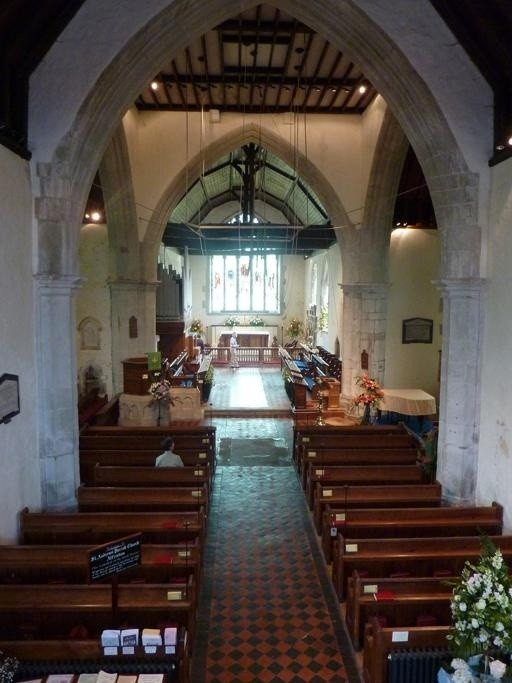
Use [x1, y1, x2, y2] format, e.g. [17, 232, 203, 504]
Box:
[229, 332, 239, 368]
[155, 438, 184, 467]
[197, 335, 205, 354]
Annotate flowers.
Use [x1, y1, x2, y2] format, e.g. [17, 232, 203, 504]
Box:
[444, 527, 511, 651]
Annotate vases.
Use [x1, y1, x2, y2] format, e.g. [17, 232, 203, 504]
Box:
[473, 635, 507, 675]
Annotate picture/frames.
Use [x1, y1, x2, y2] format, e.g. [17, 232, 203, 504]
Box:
[402, 316, 434, 343]
[77, 315, 102, 350]
[0, 372, 20, 423]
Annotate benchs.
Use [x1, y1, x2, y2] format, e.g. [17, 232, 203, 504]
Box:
[173, 363, 194, 386]
[0, 424, 216, 682]
[292, 420, 512, 682]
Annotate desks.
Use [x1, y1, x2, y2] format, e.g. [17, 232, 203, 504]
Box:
[221, 331, 268, 361]
